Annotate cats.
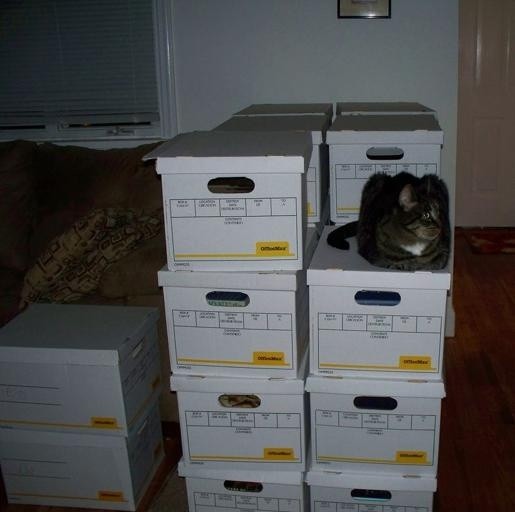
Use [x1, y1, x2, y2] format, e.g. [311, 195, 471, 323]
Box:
[327, 168, 452, 273]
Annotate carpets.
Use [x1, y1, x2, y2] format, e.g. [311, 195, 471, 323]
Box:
[458, 227, 514, 257]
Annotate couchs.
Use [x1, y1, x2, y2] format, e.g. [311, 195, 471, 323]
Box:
[0, 139, 182, 426]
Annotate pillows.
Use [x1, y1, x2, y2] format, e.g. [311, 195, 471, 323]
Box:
[10, 206, 166, 311]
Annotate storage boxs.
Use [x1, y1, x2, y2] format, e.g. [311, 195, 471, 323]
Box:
[141, 102, 452, 512]
[0, 301, 168, 512]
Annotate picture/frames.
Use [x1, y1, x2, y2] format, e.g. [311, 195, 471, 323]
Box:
[336, 0, 393, 20]
